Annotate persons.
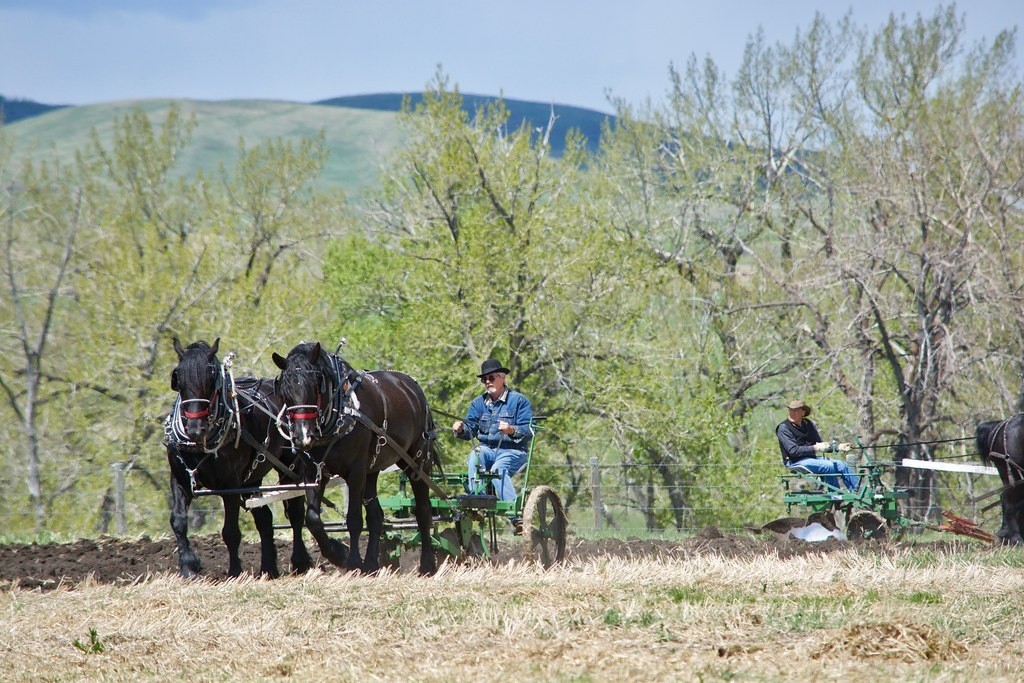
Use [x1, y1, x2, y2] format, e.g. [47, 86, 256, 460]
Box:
[453, 359, 536, 536]
[776, 400, 861, 510]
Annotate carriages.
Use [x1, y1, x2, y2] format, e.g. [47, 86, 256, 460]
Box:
[766, 410, 1024, 546]
[161, 334, 568, 579]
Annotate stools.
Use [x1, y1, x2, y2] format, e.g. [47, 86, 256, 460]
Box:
[776, 473, 819, 497]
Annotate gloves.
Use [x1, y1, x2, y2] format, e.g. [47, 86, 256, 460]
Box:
[837, 443, 852, 452]
[816, 442, 831, 452]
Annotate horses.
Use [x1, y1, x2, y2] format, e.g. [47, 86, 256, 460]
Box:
[271, 343, 447, 577]
[166, 334, 317, 580]
[975, 360, 1024, 547]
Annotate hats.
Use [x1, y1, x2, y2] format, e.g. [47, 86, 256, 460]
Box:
[476, 359, 510, 378]
[785, 399, 811, 417]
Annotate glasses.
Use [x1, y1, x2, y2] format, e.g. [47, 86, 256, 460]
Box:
[481, 375, 499, 383]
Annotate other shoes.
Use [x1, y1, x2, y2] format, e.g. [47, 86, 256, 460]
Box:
[513, 521, 523, 535]
[472, 510, 486, 524]
[835, 502, 849, 510]
